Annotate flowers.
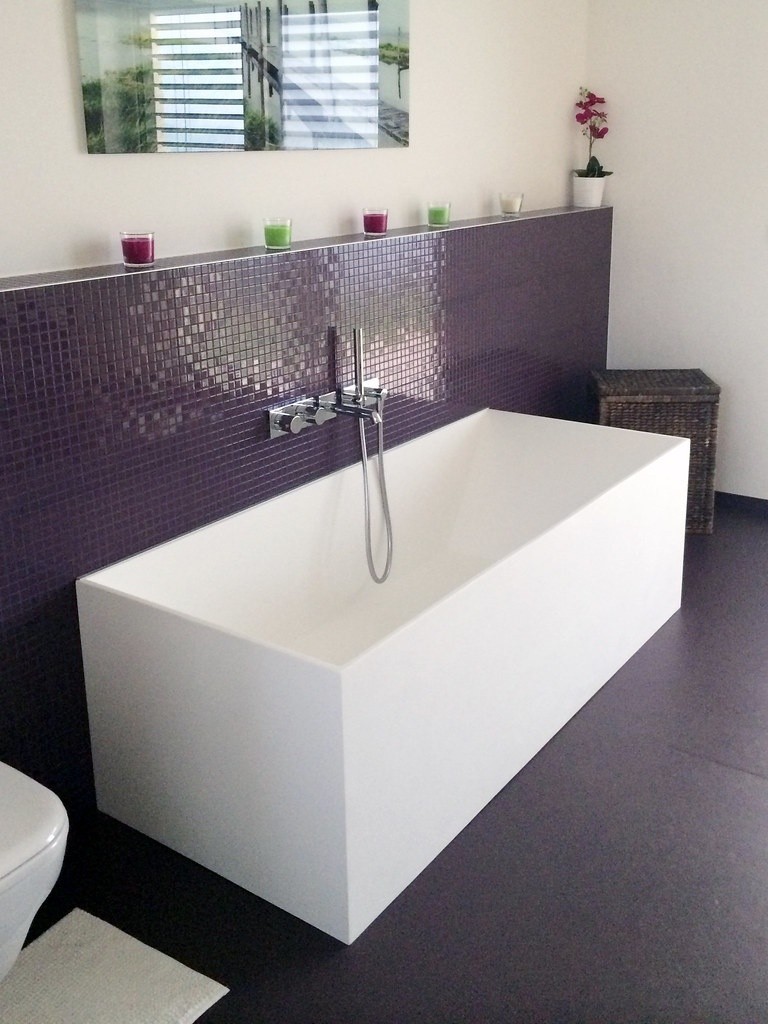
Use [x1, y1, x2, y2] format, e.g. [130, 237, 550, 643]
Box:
[574, 86, 613, 177]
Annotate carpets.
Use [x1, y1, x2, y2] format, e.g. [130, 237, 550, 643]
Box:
[0, 906, 230, 1024]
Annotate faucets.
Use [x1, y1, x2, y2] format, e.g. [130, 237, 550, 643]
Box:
[330, 402, 382, 426]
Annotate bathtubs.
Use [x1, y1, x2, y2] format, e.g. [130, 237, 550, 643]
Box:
[72, 404, 694, 947]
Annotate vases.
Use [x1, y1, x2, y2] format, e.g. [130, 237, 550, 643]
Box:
[573, 176, 606, 208]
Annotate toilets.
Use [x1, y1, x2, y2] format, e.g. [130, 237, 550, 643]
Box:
[1, 758, 72, 984]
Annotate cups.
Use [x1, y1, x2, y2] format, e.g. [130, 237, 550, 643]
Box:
[262, 217, 292, 250]
[119, 230, 155, 267]
[427, 201, 452, 227]
[499, 191, 524, 218]
[362, 207, 389, 236]
